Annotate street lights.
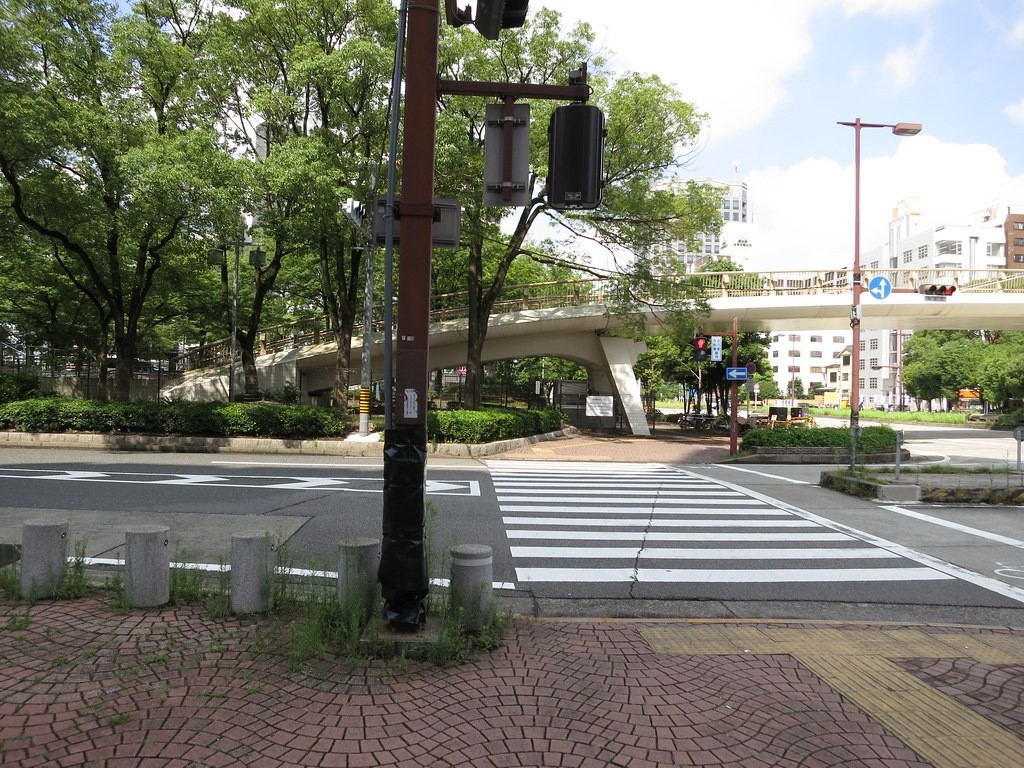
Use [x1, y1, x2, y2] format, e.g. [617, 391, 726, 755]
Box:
[206, 239, 275, 403]
[837, 118, 923, 461]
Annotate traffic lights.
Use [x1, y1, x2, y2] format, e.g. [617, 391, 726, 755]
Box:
[920, 283, 955, 297]
[694, 336, 708, 362]
[472, 0, 530, 41]
